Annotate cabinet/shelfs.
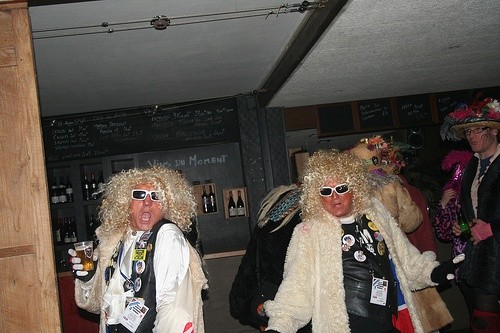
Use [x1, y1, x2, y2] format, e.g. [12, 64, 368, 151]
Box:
[46, 154, 139, 278]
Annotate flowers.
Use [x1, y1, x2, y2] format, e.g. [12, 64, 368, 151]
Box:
[360, 132, 423, 175]
[440, 97, 500, 141]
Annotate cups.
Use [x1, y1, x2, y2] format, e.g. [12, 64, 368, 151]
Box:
[74, 240, 95, 271]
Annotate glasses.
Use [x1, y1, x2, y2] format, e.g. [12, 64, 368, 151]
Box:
[465, 126, 490, 136]
[131, 189, 166, 202]
[318, 183, 349, 197]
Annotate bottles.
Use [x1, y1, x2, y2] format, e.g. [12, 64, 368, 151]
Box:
[229, 190, 245, 218]
[55, 216, 78, 244]
[89, 214, 96, 237]
[458, 209, 472, 240]
[50, 175, 73, 204]
[83, 170, 104, 201]
[201, 185, 217, 213]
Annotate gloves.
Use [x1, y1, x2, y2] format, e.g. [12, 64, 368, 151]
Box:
[68, 249, 98, 281]
[431, 253, 465, 285]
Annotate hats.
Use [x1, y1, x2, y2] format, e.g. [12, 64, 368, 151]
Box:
[349, 135, 405, 189]
[440, 97, 500, 141]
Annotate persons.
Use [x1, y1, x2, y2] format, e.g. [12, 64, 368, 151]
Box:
[229, 97, 500, 333]
[68, 159, 209, 333]
[263, 148, 465, 333]
